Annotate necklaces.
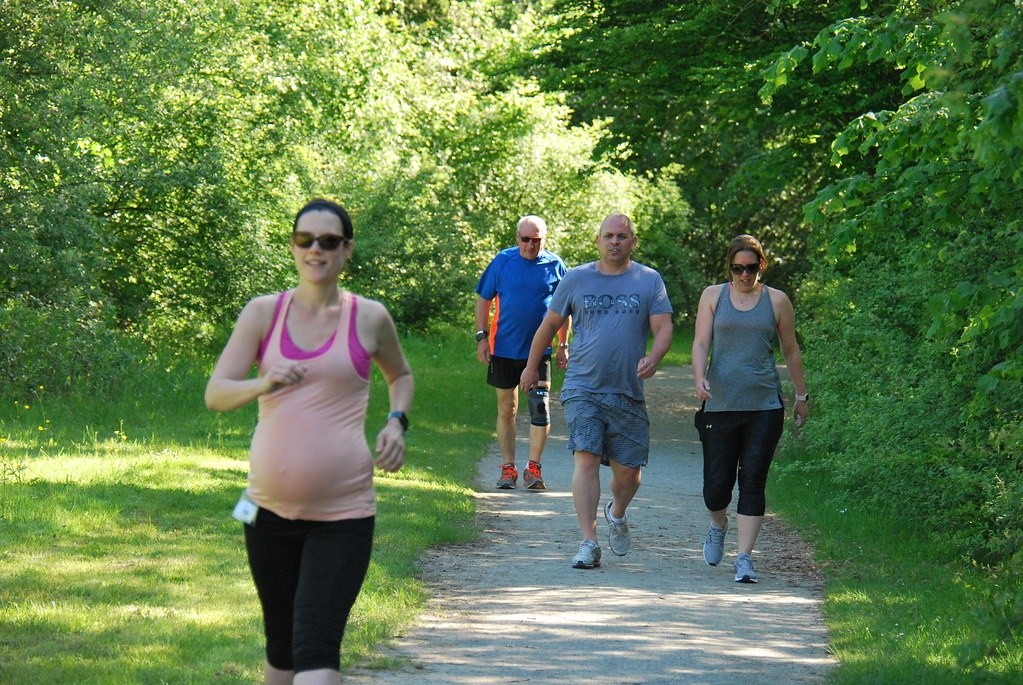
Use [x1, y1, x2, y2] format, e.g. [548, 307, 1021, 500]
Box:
[737, 292, 751, 307]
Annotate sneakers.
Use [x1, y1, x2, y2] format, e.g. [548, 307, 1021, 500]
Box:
[703, 515, 729, 566]
[734, 554, 758, 584]
[497, 462, 519, 491]
[523, 459, 546, 490]
[572, 539, 602, 569]
[604, 501, 630, 557]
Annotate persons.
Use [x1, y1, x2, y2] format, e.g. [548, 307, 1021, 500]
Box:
[204, 197, 413, 685]
[692, 233, 809, 583]
[474, 216, 572, 493]
[519, 212, 674, 570]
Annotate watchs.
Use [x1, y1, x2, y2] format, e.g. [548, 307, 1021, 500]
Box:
[388, 411, 408, 431]
[795, 393, 809, 402]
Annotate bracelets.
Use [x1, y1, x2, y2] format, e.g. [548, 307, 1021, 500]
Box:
[476, 330, 488, 341]
[560, 342, 569, 348]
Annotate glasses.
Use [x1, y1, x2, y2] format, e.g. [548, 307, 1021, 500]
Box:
[729, 263, 763, 275]
[518, 230, 544, 244]
[291, 232, 349, 251]
